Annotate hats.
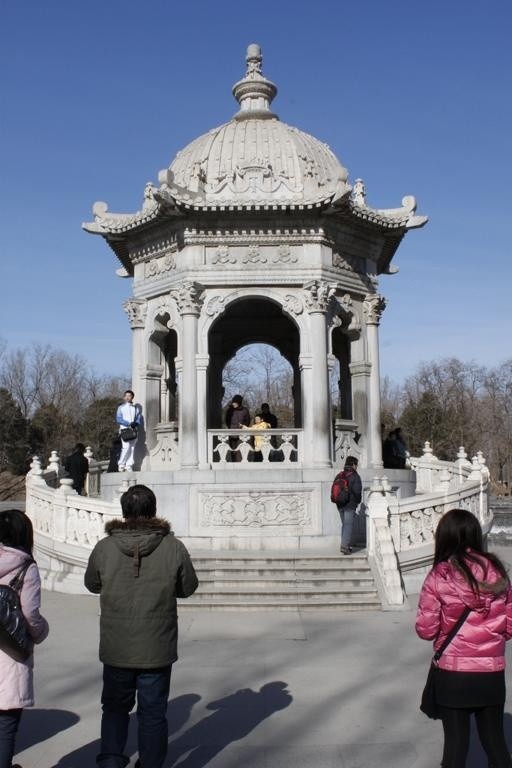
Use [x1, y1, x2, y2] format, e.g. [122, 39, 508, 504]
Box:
[232, 394, 243, 405]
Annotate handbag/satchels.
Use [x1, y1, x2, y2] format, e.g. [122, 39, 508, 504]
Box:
[0, 584, 34, 657]
[419, 664, 441, 720]
[121, 426, 137, 441]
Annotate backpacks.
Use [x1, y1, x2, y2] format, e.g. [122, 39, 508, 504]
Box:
[331, 470, 356, 504]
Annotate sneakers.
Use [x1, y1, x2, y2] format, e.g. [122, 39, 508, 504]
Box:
[118, 464, 133, 472]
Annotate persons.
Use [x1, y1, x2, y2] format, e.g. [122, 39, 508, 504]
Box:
[107, 438, 121, 472]
[114, 388, 142, 472]
[412, 507, 512, 766]
[381, 430, 404, 468]
[381, 422, 386, 440]
[225, 393, 251, 462]
[237, 413, 272, 462]
[394, 427, 406, 469]
[255, 401, 278, 461]
[0, 507, 50, 768]
[81, 483, 201, 767]
[64, 442, 90, 494]
[333, 455, 364, 556]
